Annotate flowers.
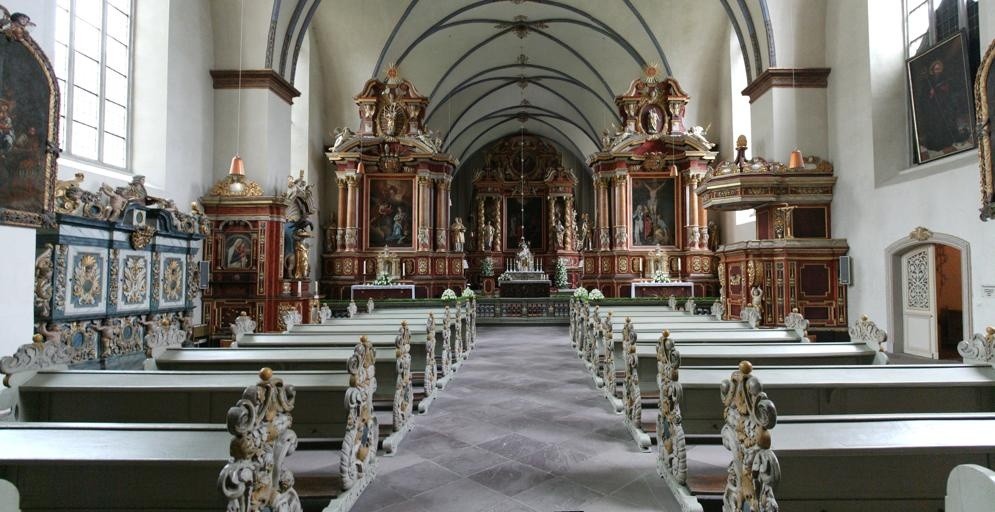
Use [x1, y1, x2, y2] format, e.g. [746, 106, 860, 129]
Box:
[651, 269, 671, 284]
[588, 288, 604, 300]
[574, 286, 588, 297]
[441, 288, 458, 302]
[461, 286, 476, 298]
[372, 271, 396, 286]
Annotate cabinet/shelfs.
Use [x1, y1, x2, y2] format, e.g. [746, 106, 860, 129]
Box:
[499, 279, 551, 298]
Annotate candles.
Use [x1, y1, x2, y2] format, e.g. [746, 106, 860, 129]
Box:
[363, 260, 366, 274]
[537, 257, 539, 264]
[677, 258, 682, 271]
[513, 258, 516, 265]
[510, 258, 512, 265]
[507, 258, 508, 264]
[639, 258, 643, 272]
[402, 262, 406, 276]
[314, 280, 319, 296]
[540, 257, 543, 264]
[297, 280, 303, 298]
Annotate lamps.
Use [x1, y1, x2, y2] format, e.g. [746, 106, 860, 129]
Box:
[785, 0, 808, 170]
[227, 0, 248, 178]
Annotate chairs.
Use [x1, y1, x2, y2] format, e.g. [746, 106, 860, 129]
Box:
[15, 366, 307, 512]
[654, 323, 995, 512]
[346, 298, 472, 361]
[582, 298, 726, 370]
[281, 305, 453, 390]
[317, 300, 465, 374]
[590, 302, 762, 389]
[576, 296, 698, 360]
[1, 335, 382, 512]
[620, 313, 890, 450]
[568, 293, 678, 349]
[365, 294, 477, 350]
[718, 358, 995, 512]
[229, 312, 440, 418]
[600, 306, 812, 413]
[137, 320, 415, 456]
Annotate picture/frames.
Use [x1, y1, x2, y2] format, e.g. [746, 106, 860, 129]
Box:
[221, 229, 253, 270]
[904, 25, 977, 166]
[502, 193, 547, 254]
[973, 36, 995, 222]
[1, 1, 65, 232]
[624, 170, 684, 253]
[362, 172, 419, 254]
